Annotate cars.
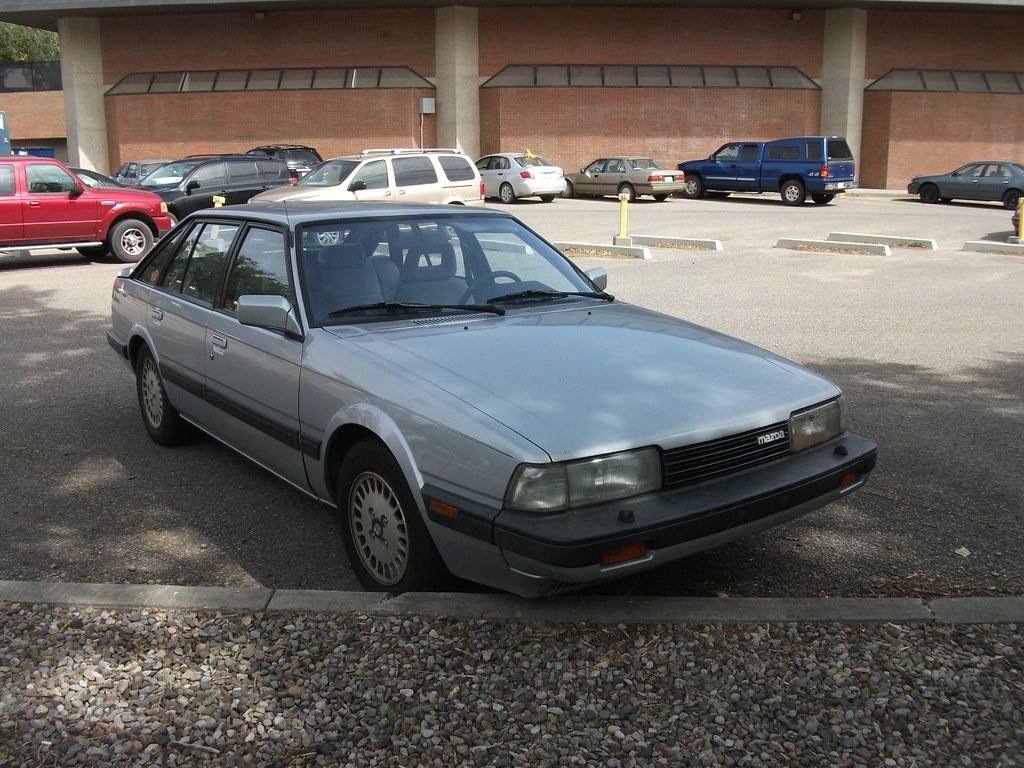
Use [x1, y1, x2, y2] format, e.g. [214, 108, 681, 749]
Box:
[0, 155, 180, 263]
[560, 156, 687, 199]
[114, 143, 326, 233]
[474, 152, 567, 204]
[907, 160, 1024, 210]
[108, 200, 880, 598]
[248, 148, 486, 207]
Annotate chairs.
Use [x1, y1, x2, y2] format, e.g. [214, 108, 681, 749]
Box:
[306, 243, 385, 323]
[495, 162, 504, 168]
[393, 242, 475, 305]
[608, 166, 619, 173]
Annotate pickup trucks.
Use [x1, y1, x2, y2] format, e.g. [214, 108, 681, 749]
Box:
[676, 134, 859, 207]
